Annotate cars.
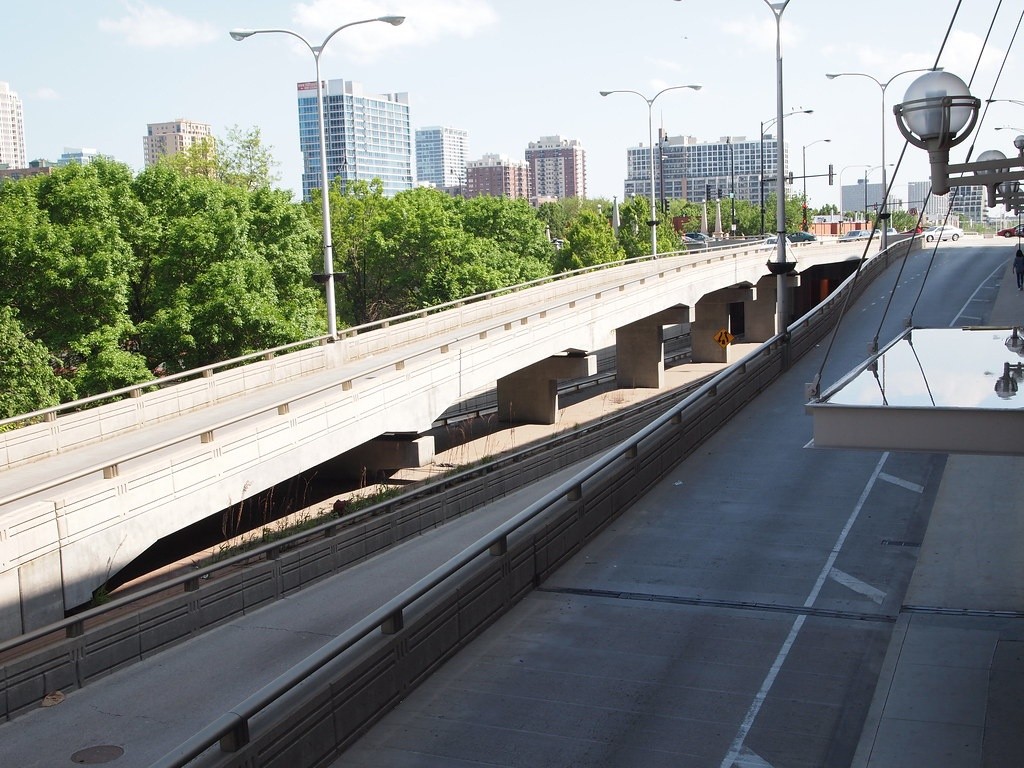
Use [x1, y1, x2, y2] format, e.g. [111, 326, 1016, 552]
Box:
[886, 228, 897, 235]
[763, 237, 792, 252]
[995, 223, 1024, 238]
[900, 226, 931, 233]
[872, 230, 882, 239]
[921, 225, 964, 243]
[681, 232, 712, 244]
[787, 232, 817, 242]
[839, 231, 871, 242]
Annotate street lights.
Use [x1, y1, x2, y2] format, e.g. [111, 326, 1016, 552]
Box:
[227, 14, 406, 342]
[599, 85, 703, 262]
[802, 138, 833, 234]
[826, 66, 944, 247]
[838, 164, 871, 234]
[725, 136, 736, 238]
[864, 163, 895, 232]
[759, 109, 816, 237]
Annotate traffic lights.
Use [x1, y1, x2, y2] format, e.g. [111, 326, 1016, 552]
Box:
[705, 183, 711, 202]
[801, 204, 807, 224]
[717, 187, 723, 200]
[663, 199, 670, 213]
